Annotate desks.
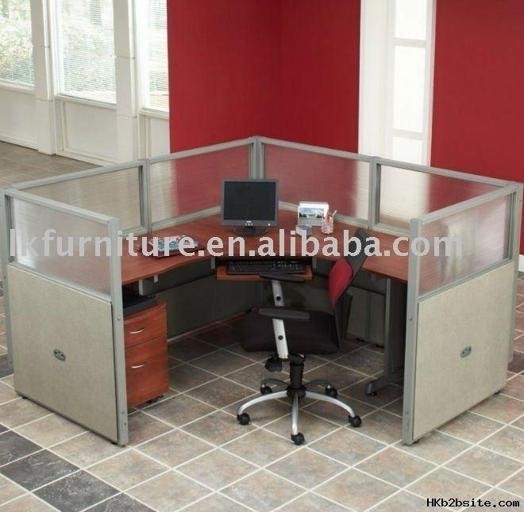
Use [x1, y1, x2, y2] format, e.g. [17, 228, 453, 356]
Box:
[38, 204, 486, 414]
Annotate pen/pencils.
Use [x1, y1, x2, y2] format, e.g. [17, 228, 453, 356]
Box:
[322, 209, 337, 222]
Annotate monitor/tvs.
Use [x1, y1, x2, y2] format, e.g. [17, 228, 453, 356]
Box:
[220, 179, 278, 236]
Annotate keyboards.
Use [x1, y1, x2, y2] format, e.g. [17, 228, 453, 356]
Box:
[226, 259, 307, 275]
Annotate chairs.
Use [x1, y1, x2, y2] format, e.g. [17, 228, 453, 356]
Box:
[237, 227, 375, 445]
